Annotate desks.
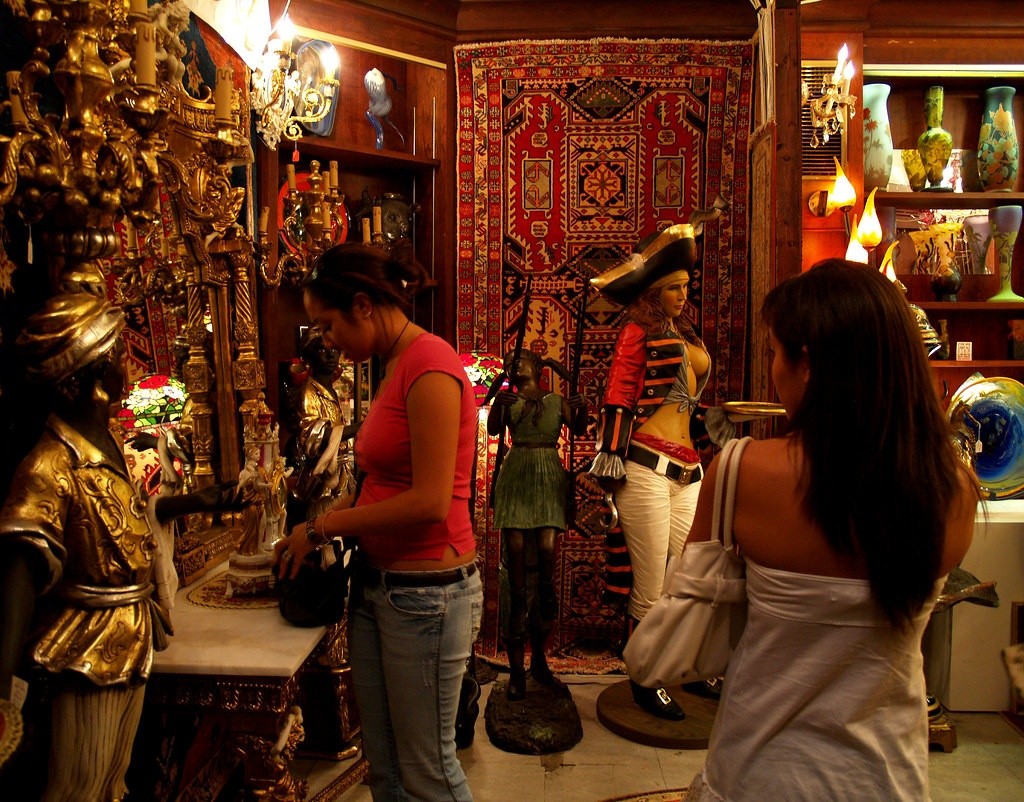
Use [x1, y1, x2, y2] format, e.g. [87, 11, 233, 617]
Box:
[136, 558, 363, 802]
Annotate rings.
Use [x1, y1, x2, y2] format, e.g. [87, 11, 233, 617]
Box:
[282, 552, 292, 559]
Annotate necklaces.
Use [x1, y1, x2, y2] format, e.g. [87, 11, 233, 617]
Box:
[670, 322, 691, 367]
[384, 321, 409, 357]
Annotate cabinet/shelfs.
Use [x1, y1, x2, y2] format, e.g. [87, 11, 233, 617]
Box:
[862, 62, 1024, 715]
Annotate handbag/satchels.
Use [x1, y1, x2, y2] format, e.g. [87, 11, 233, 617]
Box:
[622, 436, 752, 688]
[272, 541, 347, 629]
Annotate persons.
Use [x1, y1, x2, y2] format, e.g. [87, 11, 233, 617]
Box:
[273, 442, 295, 537]
[587, 222, 713, 722]
[480, 348, 587, 699]
[292, 325, 364, 569]
[677, 258, 982, 802]
[273, 238, 482, 802]
[235, 444, 274, 556]
[0, 292, 253, 802]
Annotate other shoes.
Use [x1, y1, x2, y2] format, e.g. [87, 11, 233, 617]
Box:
[530, 663, 559, 693]
[628, 677, 685, 722]
[507, 681, 537, 710]
[684, 678, 722, 701]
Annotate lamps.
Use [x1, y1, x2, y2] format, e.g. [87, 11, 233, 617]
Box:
[809, 43, 857, 148]
[827, 153, 901, 283]
[250, 10, 341, 152]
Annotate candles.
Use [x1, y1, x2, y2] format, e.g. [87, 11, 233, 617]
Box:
[286, 164, 297, 190]
[259, 206, 270, 232]
[320, 201, 331, 228]
[330, 160, 338, 185]
[361, 217, 371, 243]
[373, 206, 382, 232]
[322, 171, 331, 200]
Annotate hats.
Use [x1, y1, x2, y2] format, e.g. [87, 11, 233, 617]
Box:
[589, 223, 695, 308]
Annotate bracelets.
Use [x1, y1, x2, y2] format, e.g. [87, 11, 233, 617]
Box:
[322, 510, 335, 545]
[306, 515, 321, 544]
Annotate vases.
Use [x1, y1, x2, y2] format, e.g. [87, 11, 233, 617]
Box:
[987, 205, 1024, 304]
[918, 86, 954, 191]
[862, 84, 893, 193]
[977, 86, 1019, 191]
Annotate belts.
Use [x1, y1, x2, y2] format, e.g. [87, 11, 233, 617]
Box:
[626, 446, 701, 485]
[375, 564, 477, 587]
